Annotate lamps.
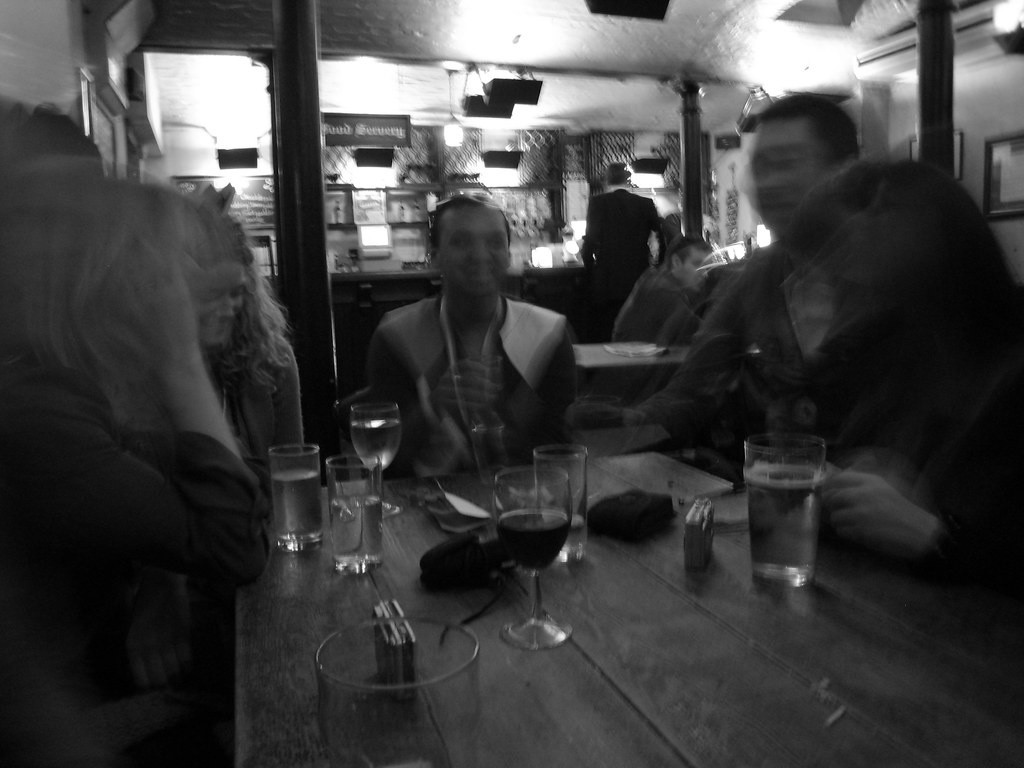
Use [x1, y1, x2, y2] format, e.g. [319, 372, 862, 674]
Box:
[443, 68, 463, 146]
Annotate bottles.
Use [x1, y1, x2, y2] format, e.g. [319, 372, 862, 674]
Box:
[415, 199, 420, 221]
[336, 201, 342, 223]
[399, 200, 404, 222]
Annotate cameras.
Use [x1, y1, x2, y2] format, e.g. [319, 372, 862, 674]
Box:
[682, 497, 714, 571]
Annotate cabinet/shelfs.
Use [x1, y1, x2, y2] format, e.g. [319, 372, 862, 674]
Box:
[322, 125, 565, 272]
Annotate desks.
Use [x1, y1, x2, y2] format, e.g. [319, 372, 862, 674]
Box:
[236, 453, 1024, 768]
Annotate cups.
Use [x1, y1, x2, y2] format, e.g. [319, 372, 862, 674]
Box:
[533, 444, 589, 561]
[269, 443, 324, 553]
[744, 434, 827, 587]
[325, 453, 384, 575]
[313, 616, 481, 767]
[467, 355, 505, 433]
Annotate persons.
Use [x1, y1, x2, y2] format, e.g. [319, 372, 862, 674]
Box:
[192, 182, 304, 479]
[0, 95, 263, 767]
[566, 88, 1024, 644]
[367, 190, 575, 481]
[612, 233, 711, 343]
[580, 162, 666, 342]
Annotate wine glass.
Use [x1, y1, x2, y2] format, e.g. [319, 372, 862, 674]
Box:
[350, 402, 403, 518]
[492, 468, 572, 649]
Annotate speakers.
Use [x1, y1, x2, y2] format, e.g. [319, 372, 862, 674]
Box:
[353, 147, 394, 167]
[461, 78, 543, 118]
[632, 158, 668, 174]
[218, 149, 258, 170]
[584, 0, 669, 22]
[484, 151, 521, 168]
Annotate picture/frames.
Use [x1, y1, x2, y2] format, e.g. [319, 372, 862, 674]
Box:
[982, 131, 1024, 220]
[910, 130, 962, 180]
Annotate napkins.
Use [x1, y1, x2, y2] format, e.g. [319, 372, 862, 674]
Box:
[587, 489, 676, 542]
